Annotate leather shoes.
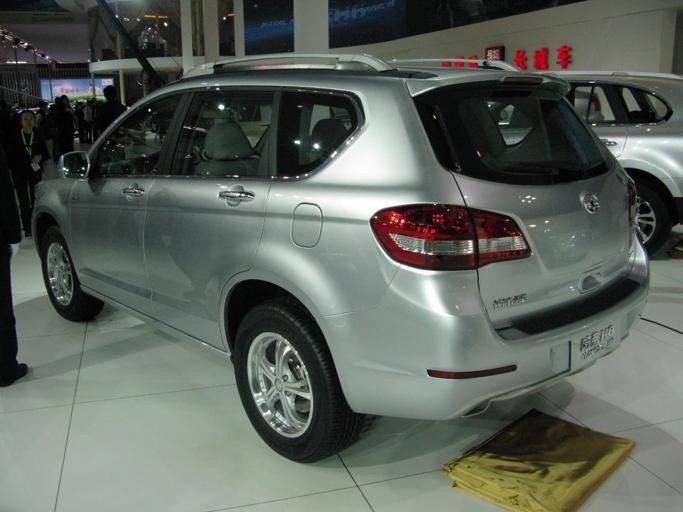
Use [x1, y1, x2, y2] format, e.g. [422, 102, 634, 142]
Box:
[0, 364, 27, 387]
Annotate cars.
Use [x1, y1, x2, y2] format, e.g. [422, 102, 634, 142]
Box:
[29, 50, 649, 465]
[328, 70, 682, 260]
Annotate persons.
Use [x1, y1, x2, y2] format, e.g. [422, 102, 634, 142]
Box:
[0, 88, 28, 388]
[10, 96, 103, 162]
[11, 110, 44, 237]
[96, 85, 126, 132]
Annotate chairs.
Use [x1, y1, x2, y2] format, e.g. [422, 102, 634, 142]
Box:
[196, 122, 261, 175]
[308, 115, 347, 167]
